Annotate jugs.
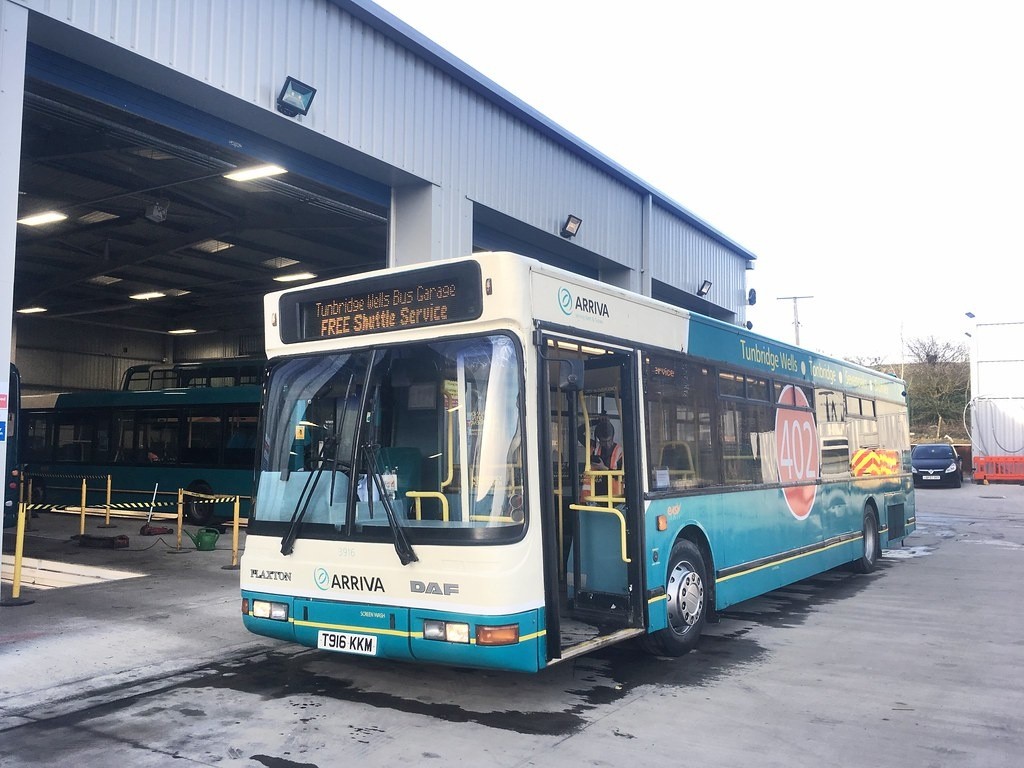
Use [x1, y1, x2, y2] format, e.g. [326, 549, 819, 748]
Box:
[184, 528, 219, 550]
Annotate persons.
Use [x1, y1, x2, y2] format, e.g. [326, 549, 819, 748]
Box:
[577, 416, 623, 507]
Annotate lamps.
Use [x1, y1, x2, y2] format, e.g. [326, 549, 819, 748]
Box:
[560, 214, 582, 238]
[697, 280, 712, 296]
[277, 76, 317, 117]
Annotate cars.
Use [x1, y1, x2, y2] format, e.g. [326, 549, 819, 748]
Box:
[912, 443, 965, 489]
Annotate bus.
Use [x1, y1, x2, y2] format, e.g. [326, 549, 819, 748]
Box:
[239, 251, 916, 675]
[3, 355, 267, 529]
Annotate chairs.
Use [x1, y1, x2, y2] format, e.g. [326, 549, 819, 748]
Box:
[373, 441, 761, 530]
